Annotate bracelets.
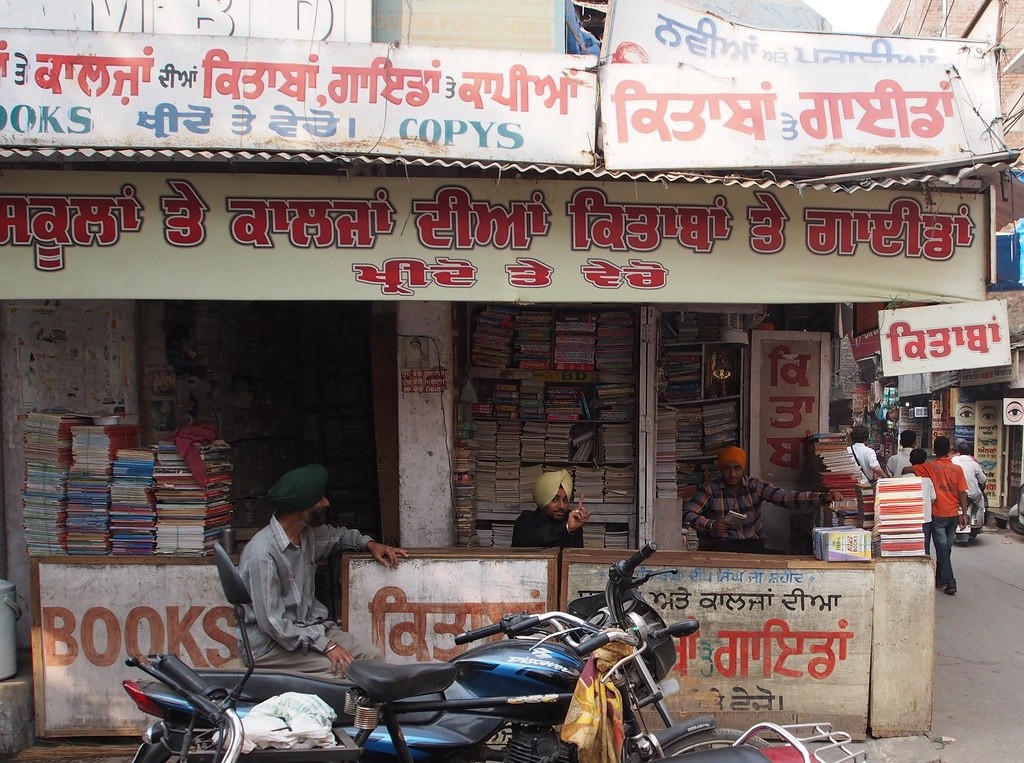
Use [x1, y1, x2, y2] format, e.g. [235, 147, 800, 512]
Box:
[322, 642, 339, 653]
[819, 493, 828, 506]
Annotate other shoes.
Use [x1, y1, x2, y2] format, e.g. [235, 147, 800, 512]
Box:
[936, 578, 947, 588]
[944, 586, 957, 594]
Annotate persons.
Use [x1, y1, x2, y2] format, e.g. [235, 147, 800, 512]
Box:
[901, 436, 969, 594]
[947, 438, 986, 534]
[511, 468, 592, 548]
[682, 445, 843, 555]
[235, 465, 409, 679]
[846, 426, 887, 483]
[886, 430, 917, 478]
[909, 449, 937, 555]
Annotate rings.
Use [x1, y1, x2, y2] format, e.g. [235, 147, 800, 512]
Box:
[575, 509, 579, 513]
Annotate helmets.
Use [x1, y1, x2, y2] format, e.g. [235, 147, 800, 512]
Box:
[958, 441, 971, 454]
[952, 438, 966, 450]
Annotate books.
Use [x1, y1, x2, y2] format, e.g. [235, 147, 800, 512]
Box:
[656, 312, 740, 499]
[21, 412, 234, 557]
[724, 510, 747, 529]
[682, 522, 698, 552]
[453, 304, 634, 549]
[147, 300, 383, 530]
[806, 432, 925, 561]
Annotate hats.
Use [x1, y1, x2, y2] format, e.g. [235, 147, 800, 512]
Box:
[267, 464, 327, 512]
[531, 469, 573, 509]
[717, 446, 746, 471]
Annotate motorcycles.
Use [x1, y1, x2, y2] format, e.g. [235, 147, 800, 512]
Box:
[126, 536, 871, 763]
[1008, 485, 1024, 535]
[954, 471, 988, 547]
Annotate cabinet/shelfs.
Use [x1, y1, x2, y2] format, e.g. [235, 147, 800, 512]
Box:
[457, 305, 750, 549]
